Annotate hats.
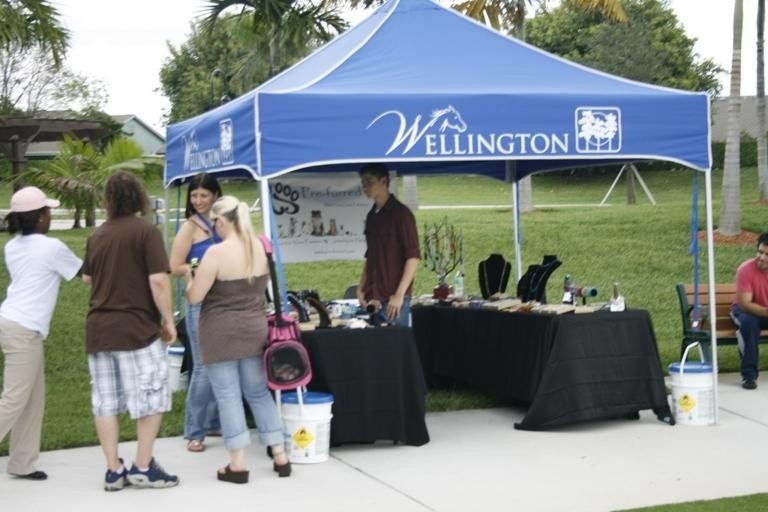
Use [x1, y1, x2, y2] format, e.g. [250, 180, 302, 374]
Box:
[10, 185, 61, 213]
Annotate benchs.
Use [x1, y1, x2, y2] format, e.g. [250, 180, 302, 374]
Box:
[675, 282, 768, 364]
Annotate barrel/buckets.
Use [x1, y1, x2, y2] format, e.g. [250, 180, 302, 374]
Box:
[166, 346, 189, 392]
[278, 382, 334, 464]
[667, 340, 713, 426]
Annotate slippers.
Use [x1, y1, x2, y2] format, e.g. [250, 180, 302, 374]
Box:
[273, 460, 291, 476]
[218, 464, 249, 483]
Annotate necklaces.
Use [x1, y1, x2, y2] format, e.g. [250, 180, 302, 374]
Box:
[483, 260, 507, 297]
[529, 260, 558, 292]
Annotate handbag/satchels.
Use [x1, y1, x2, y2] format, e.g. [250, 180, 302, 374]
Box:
[264, 314, 312, 391]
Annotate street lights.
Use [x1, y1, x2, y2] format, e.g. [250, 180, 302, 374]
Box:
[209, 67, 232, 112]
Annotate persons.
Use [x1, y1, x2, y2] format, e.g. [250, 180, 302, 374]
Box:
[732, 232, 768, 388]
[169, 172, 221, 452]
[80, 169, 179, 491]
[0, 186, 82, 481]
[356, 162, 422, 326]
[183, 195, 291, 482]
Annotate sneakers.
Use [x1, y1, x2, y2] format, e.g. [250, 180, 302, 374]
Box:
[125, 457, 180, 488]
[105, 457, 131, 491]
[8, 470, 47, 480]
[186, 439, 205, 452]
[741, 376, 756, 389]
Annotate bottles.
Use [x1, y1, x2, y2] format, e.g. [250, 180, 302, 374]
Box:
[453, 271, 465, 301]
